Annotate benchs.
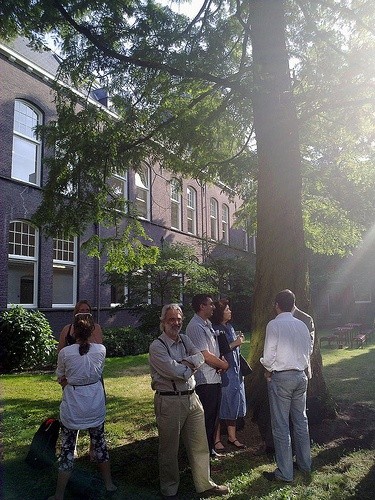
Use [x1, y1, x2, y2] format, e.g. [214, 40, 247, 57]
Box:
[320, 335, 335, 348]
[353, 329, 373, 349]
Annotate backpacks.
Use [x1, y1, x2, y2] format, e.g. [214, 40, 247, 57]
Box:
[24, 418, 61, 471]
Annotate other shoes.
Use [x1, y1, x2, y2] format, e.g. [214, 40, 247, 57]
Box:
[262, 471, 292, 483]
[213, 440, 227, 453]
[227, 438, 247, 449]
[43, 494, 58, 500]
[104, 480, 120, 498]
[293, 461, 300, 469]
[162, 495, 179, 500]
[198, 483, 229, 495]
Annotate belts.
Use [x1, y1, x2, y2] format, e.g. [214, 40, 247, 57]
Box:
[155, 389, 195, 396]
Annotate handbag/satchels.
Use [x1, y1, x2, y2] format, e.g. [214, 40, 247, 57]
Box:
[217, 332, 231, 355]
[240, 353, 252, 376]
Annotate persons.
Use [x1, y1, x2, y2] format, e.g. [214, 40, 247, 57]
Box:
[46, 299, 116, 500]
[211, 298, 246, 452]
[149, 303, 228, 500]
[260, 288, 315, 481]
[185, 293, 229, 457]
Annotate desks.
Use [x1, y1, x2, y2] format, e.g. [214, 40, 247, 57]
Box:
[333, 321, 362, 349]
